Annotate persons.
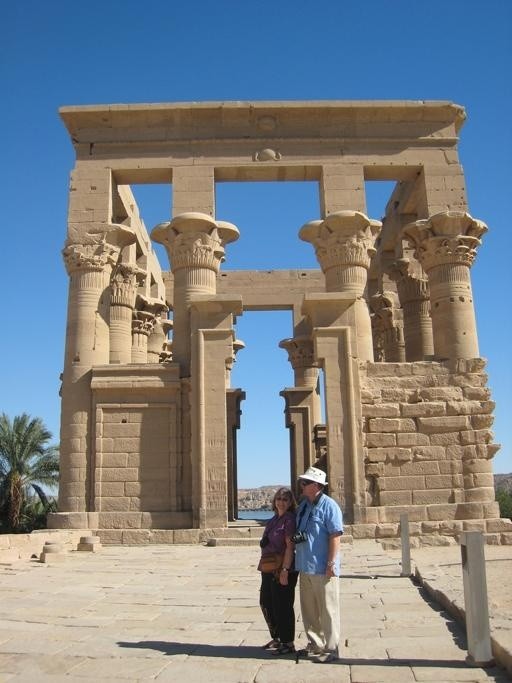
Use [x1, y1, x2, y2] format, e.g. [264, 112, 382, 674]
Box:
[257, 487, 299, 656]
[295, 466, 345, 663]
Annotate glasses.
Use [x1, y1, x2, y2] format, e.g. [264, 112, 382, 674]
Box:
[277, 497, 290, 502]
[303, 480, 313, 486]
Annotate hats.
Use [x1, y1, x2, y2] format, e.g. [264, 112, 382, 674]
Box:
[299, 467, 328, 486]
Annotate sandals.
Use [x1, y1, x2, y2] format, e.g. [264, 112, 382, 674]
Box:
[272, 644, 296, 655]
[264, 640, 281, 649]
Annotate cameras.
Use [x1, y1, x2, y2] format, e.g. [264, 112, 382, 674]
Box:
[292, 532, 308, 544]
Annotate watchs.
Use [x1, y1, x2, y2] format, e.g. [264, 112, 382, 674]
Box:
[282, 567, 291, 572]
[326, 560, 336, 567]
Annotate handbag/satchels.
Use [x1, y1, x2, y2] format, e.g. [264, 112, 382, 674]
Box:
[259, 553, 284, 572]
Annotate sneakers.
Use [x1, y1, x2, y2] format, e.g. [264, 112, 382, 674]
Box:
[297, 643, 338, 663]
